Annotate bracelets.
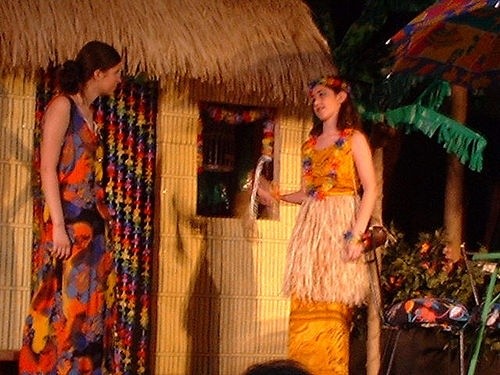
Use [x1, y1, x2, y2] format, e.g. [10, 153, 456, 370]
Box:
[344, 231, 363, 246]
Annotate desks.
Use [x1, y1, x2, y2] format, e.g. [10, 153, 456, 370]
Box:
[468, 254, 500, 375]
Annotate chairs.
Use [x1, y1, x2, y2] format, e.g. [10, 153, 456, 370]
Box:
[362, 226, 469, 375]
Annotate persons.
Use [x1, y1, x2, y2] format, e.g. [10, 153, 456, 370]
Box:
[258, 78, 379, 375]
[16, 40, 122, 375]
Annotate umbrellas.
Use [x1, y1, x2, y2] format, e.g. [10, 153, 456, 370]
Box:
[385, 0, 500, 100]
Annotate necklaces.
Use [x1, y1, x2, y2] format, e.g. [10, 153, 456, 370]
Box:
[300, 126, 356, 200]
